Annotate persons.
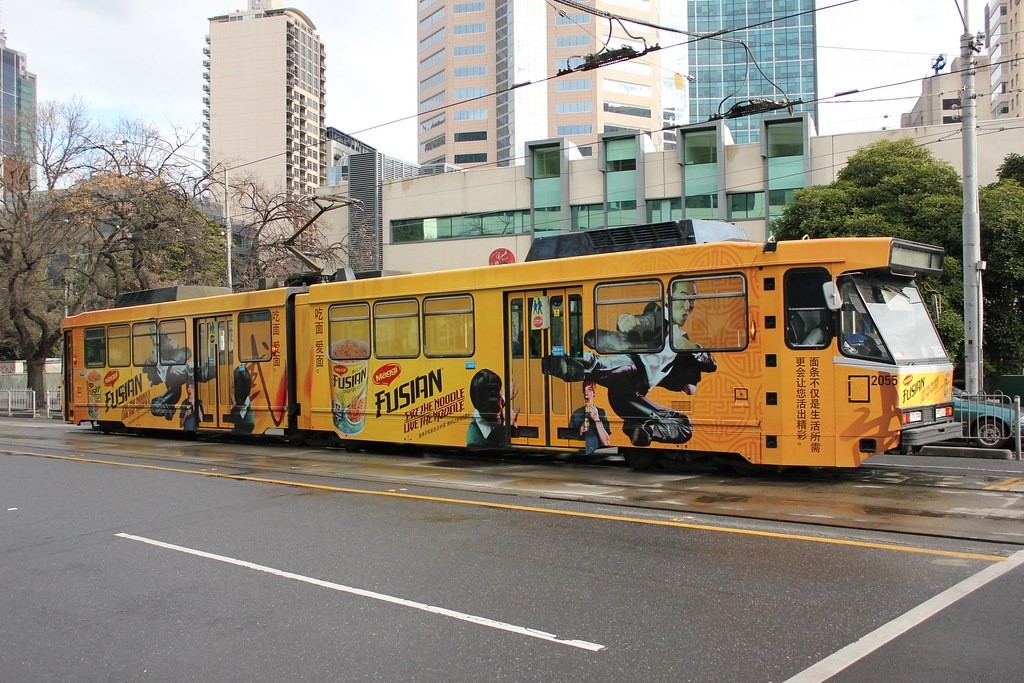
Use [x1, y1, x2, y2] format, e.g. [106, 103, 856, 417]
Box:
[802, 312, 837, 346]
[568, 378, 612, 454]
[179, 381, 205, 430]
[228, 365, 256, 433]
[142, 325, 215, 421]
[540, 275, 717, 447]
[465, 368, 521, 448]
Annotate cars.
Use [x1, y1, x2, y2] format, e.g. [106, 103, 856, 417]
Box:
[951, 382, 1024, 450]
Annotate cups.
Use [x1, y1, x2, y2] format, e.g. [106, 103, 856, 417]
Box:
[86, 370, 101, 418]
[327, 339, 370, 436]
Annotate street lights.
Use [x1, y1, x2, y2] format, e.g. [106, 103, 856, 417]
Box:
[111, 138, 233, 292]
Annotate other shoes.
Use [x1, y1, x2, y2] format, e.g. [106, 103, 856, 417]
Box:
[622, 423, 652, 446]
[541, 354, 585, 381]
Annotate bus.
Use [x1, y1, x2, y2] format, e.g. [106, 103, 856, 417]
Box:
[57, 218, 964, 480]
